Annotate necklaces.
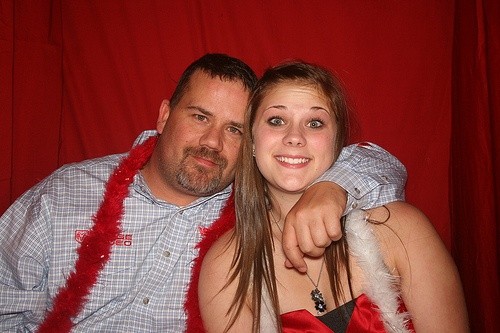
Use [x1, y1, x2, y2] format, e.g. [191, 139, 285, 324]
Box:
[269, 204, 329, 314]
[254, 203, 410, 333]
[34, 133, 237, 333]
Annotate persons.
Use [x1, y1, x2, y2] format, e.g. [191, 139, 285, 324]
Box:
[0, 50, 408, 333]
[198, 56, 473, 333]
